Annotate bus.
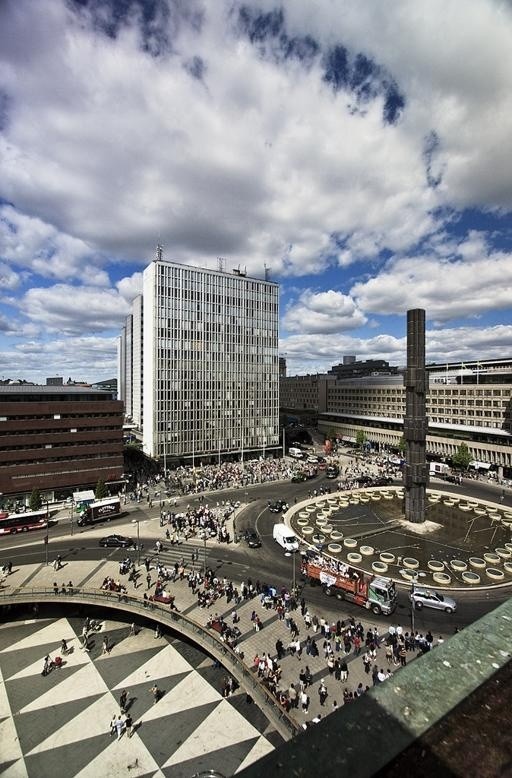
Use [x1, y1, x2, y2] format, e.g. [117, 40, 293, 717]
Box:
[0, 511, 50, 535]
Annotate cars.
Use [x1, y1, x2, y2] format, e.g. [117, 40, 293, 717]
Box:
[245, 528, 261, 548]
[353, 476, 393, 487]
[269, 499, 287, 513]
[408, 587, 457, 614]
[99, 534, 134, 548]
[289, 456, 339, 484]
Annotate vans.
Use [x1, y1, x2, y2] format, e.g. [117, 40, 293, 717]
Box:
[273, 524, 300, 553]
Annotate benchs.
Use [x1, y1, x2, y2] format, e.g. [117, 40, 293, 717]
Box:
[153, 594, 175, 603]
[208, 621, 224, 635]
[100, 584, 124, 591]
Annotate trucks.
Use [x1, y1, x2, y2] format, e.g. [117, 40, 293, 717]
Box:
[289, 447, 303, 459]
[301, 547, 399, 615]
[76, 496, 122, 527]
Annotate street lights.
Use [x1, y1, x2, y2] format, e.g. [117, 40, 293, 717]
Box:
[129, 519, 149, 567]
[398, 568, 427, 636]
[39, 495, 52, 566]
[283, 544, 307, 601]
[198, 528, 216, 578]
